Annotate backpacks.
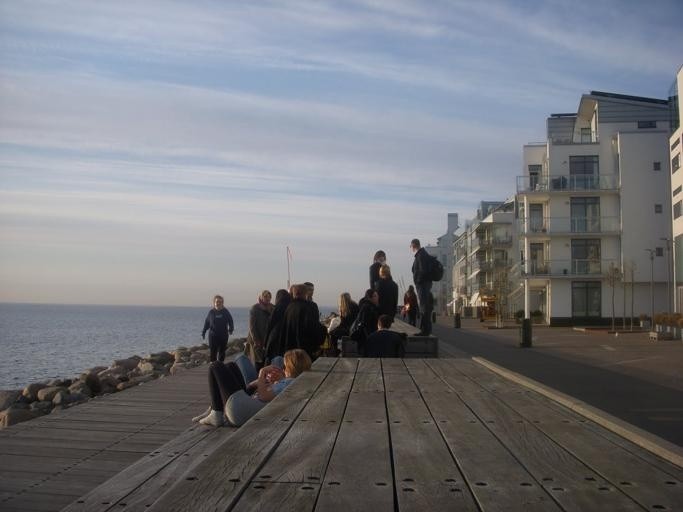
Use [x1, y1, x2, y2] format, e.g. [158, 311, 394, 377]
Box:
[426, 253, 443, 281]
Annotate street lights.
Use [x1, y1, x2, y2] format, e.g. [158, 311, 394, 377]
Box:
[661, 236, 680, 318]
[644, 247, 657, 330]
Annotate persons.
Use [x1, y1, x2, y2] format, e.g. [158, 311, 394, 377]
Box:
[192, 349, 311, 426]
[327, 250, 405, 358]
[404, 285, 420, 327]
[410, 239, 433, 336]
[249, 282, 321, 373]
[201, 295, 234, 364]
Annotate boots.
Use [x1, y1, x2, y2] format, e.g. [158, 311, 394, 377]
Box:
[191, 406, 222, 426]
